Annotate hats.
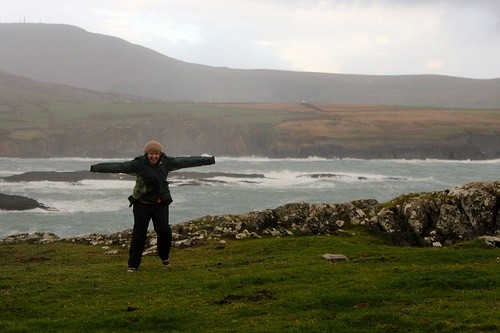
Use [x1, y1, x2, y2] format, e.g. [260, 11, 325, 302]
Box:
[143, 140, 162, 153]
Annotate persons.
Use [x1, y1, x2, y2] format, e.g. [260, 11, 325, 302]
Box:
[89, 140, 217, 272]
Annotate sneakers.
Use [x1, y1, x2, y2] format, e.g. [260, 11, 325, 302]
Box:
[162, 258, 171, 267]
[127, 267, 136, 273]
